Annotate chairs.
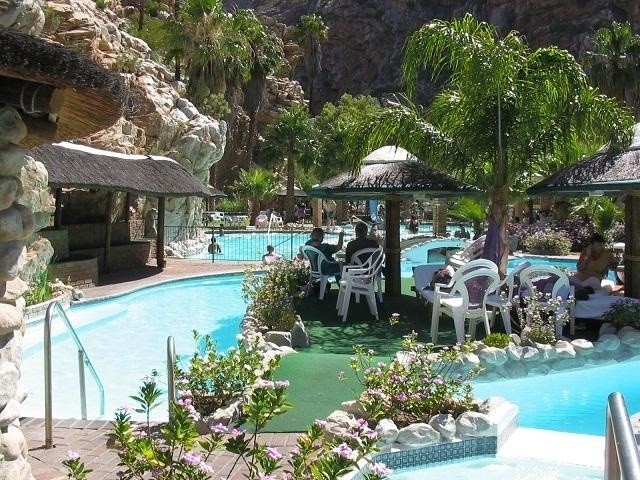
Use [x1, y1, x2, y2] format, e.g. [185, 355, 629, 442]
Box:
[301, 244, 345, 301]
[413, 231, 637, 347]
[209, 211, 285, 229]
[332, 243, 390, 325]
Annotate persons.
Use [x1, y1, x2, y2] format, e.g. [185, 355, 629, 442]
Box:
[569, 217, 622, 231]
[293, 246, 309, 262]
[262, 245, 282, 266]
[270, 200, 307, 225]
[345, 223, 383, 276]
[304, 228, 348, 289]
[568, 232, 625, 301]
[326, 200, 385, 236]
[409, 214, 419, 230]
[219, 223, 225, 236]
[208, 238, 221, 254]
[510, 209, 554, 223]
